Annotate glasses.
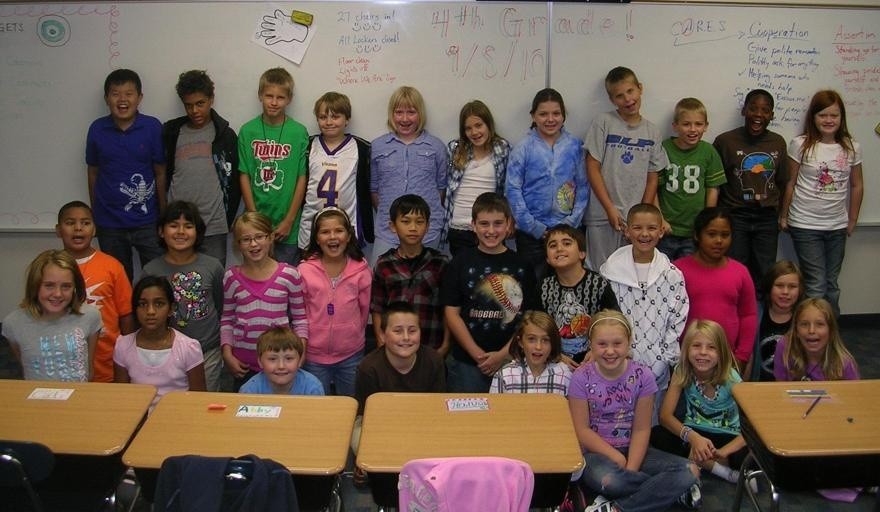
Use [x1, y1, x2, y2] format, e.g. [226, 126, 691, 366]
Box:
[238, 233, 268, 245]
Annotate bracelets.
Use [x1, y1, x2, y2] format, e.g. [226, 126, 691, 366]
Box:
[680, 424, 692, 442]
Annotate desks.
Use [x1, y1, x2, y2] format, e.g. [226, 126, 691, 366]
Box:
[728, 379, 880, 511]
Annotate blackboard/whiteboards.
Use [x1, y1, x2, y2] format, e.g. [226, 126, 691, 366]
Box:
[551, 0, 880, 226]
[0, 0, 550, 233]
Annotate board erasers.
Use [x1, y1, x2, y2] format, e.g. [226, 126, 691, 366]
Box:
[291, 10, 313, 26]
[875, 123, 880, 135]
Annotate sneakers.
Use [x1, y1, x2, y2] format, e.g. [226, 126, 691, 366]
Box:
[678, 483, 702, 510]
[558, 482, 586, 511]
[745, 470, 760, 494]
[584, 494, 615, 512]
[353, 464, 371, 492]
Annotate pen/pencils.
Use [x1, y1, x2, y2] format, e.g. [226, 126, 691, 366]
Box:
[802, 396, 821, 418]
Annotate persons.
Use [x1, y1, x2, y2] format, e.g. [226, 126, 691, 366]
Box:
[445, 97, 512, 255]
[712, 88, 788, 272]
[585, 66, 670, 277]
[506, 86, 595, 272]
[4, 196, 621, 492]
[672, 208, 759, 376]
[298, 91, 376, 256]
[650, 94, 728, 265]
[752, 259, 880, 501]
[659, 322, 758, 512]
[81, 65, 168, 278]
[566, 311, 700, 512]
[234, 64, 310, 266]
[778, 87, 864, 322]
[369, 83, 452, 260]
[156, 68, 242, 261]
[601, 205, 689, 429]
